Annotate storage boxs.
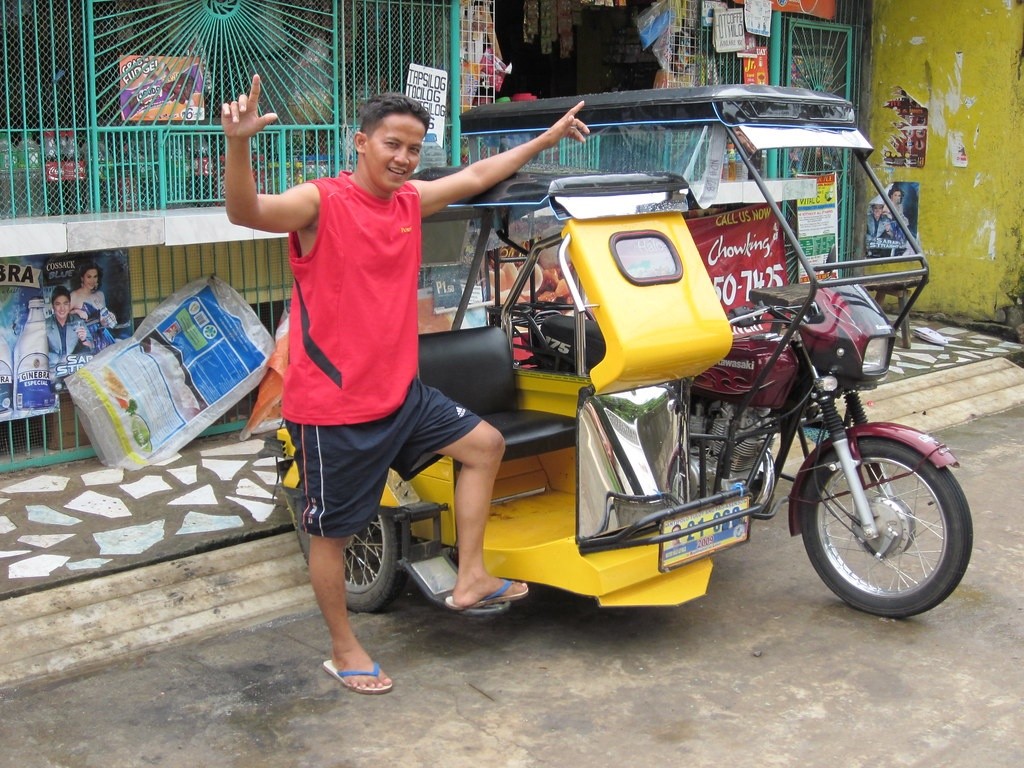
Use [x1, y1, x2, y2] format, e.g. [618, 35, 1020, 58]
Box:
[47, 394, 91, 448]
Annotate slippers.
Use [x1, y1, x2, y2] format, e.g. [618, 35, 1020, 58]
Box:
[445, 579, 529, 610]
[323, 659, 393, 694]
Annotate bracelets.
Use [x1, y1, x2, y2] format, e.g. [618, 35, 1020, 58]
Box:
[81, 339, 87, 342]
[886, 231, 889, 232]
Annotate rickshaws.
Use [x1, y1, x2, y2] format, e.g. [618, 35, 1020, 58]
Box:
[274, 85, 974, 618]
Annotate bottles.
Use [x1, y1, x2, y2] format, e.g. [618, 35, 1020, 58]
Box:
[0, 299, 57, 413]
[40, 131, 63, 216]
[417, 134, 449, 172]
[16, 131, 45, 216]
[93, 298, 117, 328]
[63, 130, 87, 215]
[0, 132, 18, 220]
[720, 134, 767, 181]
[78, 131, 367, 217]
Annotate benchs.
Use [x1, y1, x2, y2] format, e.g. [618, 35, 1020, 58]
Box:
[749, 275, 930, 350]
[418, 325, 577, 468]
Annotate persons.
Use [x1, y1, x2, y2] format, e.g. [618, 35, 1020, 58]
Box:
[46, 264, 118, 368]
[865, 186, 909, 257]
[222, 74, 590, 695]
